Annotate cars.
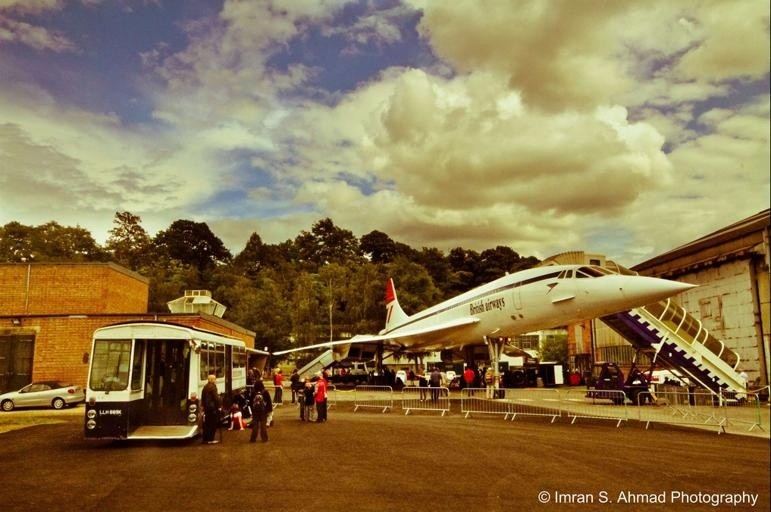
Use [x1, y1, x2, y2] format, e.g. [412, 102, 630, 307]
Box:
[633, 367, 689, 387]
[0, 378, 86, 411]
[346, 362, 569, 389]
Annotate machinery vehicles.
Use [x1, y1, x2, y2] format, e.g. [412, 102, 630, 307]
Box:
[535, 251, 747, 406]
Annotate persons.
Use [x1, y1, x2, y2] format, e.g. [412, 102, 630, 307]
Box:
[200, 373, 222, 445]
[226, 365, 496, 444]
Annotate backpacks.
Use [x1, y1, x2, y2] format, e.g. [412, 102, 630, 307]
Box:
[253, 391, 266, 411]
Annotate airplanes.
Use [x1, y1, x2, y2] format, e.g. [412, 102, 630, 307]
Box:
[268, 260, 705, 402]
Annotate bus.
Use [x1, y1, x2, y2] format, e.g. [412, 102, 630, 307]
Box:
[82, 320, 248, 446]
